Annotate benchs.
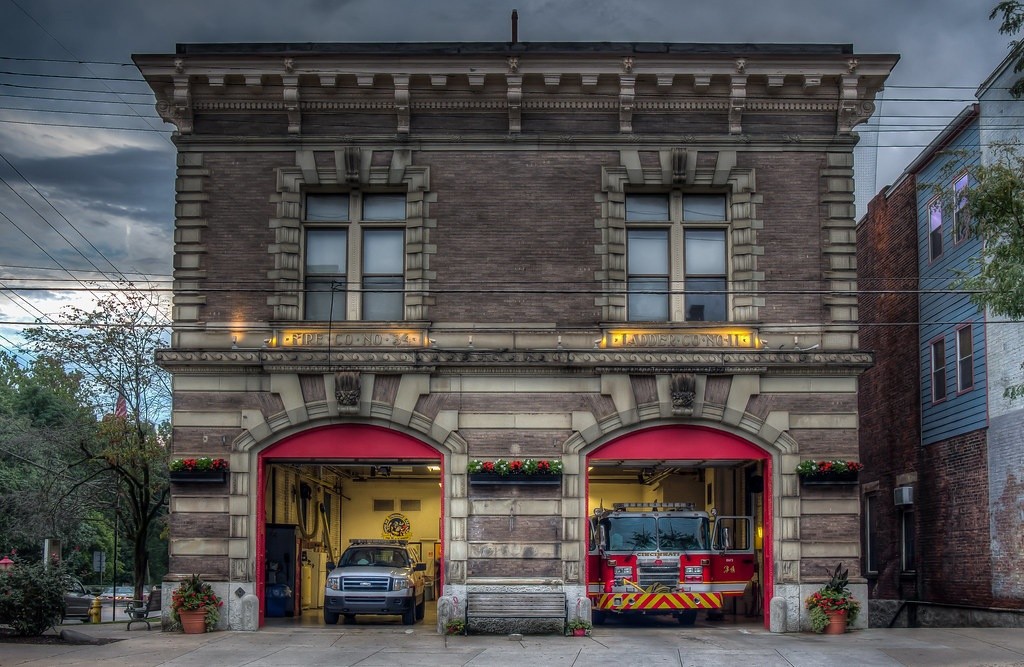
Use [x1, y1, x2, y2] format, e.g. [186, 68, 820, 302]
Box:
[125, 589, 161, 632]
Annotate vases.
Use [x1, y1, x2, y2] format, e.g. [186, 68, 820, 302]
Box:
[802, 473, 859, 488]
[446, 627, 457, 635]
[177, 606, 208, 633]
[824, 610, 847, 636]
[170, 472, 226, 483]
[574, 629, 586, 637]
[470, 473, 561, 489]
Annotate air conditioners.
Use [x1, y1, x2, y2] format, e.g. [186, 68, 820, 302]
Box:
[894, 486, 914, 507]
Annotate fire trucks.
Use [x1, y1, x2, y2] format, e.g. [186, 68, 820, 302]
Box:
[586, 499, 756, 626]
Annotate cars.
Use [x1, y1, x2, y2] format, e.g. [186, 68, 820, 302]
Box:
[323, 538, 427, 625]
[52, 577, 96, 624]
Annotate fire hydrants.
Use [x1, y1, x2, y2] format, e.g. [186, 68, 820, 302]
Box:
[86, 597, 103, 624]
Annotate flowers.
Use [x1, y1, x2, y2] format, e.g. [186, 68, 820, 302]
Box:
[172, 573, 227, 634]
[467, 461, 564, 476]
[563, 598, 594, 630]
[170, 456, 228, 472]
[794, 459, 863, 471]
[807, 562, 861, 631]
[441, 597, 463, 629]
